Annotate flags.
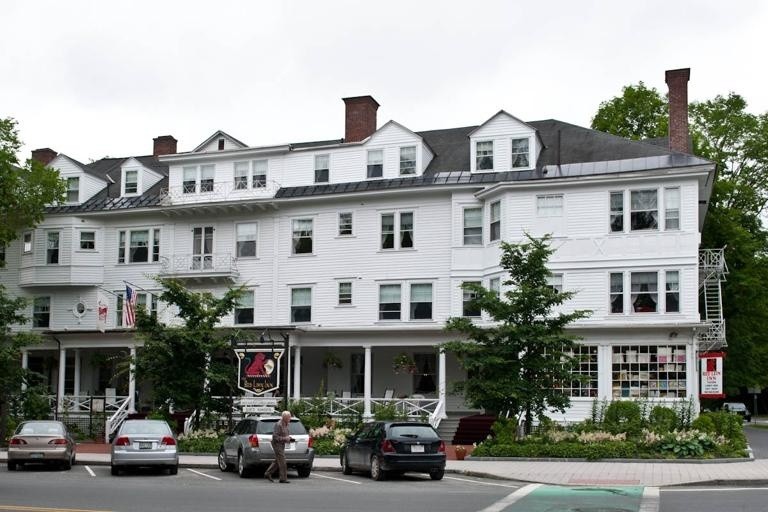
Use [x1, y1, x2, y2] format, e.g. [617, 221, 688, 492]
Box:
[96, 291, 108, 333]
[126, 285, 138, 326]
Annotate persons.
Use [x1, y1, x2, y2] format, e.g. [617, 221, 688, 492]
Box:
[264, 411, 295, 483]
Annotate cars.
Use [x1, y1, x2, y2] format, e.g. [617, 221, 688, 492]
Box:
[109, 418, 179, 475]
[4, 419, 76, 470]
[723, 402, 752, 423]
[340, 420, 447, 481]
[218, 416, 315, 478]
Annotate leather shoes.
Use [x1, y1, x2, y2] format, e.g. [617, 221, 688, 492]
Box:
[265, 472, 290, 483]
